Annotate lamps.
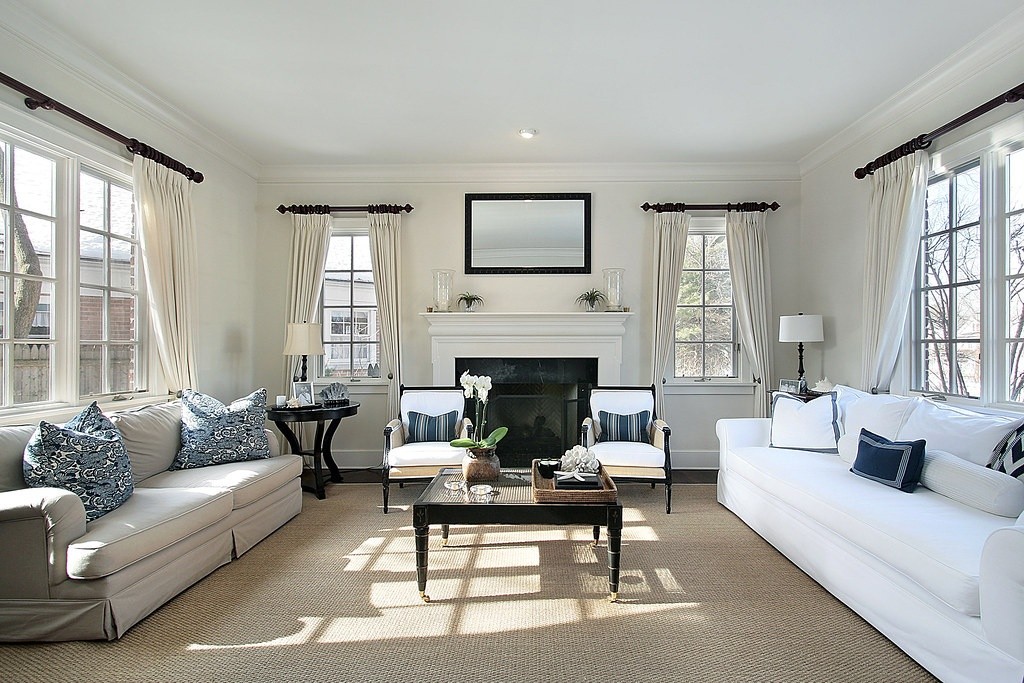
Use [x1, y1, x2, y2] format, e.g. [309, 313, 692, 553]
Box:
[281, 320, 327, 381]
[779, 312, 825, 392]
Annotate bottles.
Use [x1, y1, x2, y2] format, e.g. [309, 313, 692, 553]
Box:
[432, 268, 456, 310]
[536, 460, 560, 479]
[602, 268, 624, 312]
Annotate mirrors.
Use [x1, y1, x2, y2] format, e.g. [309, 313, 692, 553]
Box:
[464, 190, 591, 275]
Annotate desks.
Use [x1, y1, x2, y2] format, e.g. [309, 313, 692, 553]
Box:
[411, 467, 623, 604]
[266, 400, 360, 500]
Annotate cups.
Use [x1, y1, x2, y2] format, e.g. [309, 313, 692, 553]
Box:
[275, 395, 287, 405]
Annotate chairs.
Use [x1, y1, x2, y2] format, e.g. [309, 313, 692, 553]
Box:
[383, 384, 474, 514]
[580, 383, 672, 515]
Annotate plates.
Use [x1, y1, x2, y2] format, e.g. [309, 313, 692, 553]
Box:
[470, 485, 492, 495]
[444, 479, 464, 491]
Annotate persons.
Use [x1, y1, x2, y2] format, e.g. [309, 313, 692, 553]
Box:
[298, 387, 311, 404]
[781, 382, 796, 392]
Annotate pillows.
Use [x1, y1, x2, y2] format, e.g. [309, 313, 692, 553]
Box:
[849, 428, 926, 494]
[168, 386, 272, 473]
[597, 410, 653, 442]
[767, 391, 845, 453]
[22, 400, 134, 523]
[917, 450, 1024, 518]
[984, 420, 1024, 479]
[405, 410, 460, 443]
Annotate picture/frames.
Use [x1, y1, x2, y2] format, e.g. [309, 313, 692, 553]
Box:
[292, 382, 314, 407]
[779, 378, 801, 394]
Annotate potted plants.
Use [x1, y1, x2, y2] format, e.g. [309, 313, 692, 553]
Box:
[456, 291, 486, 312]
[574, 287, 609, 311]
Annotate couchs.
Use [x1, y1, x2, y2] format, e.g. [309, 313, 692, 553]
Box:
[715, 384, 1024, 683]
[0, 398, 304, 642]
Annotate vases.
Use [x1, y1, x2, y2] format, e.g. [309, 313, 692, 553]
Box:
[431, 269, 456, 312]
[462, 448, 502, 481]
[602, 267, 625, 312]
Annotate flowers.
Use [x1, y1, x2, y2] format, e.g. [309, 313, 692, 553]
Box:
[449, 368, 508, 448]
